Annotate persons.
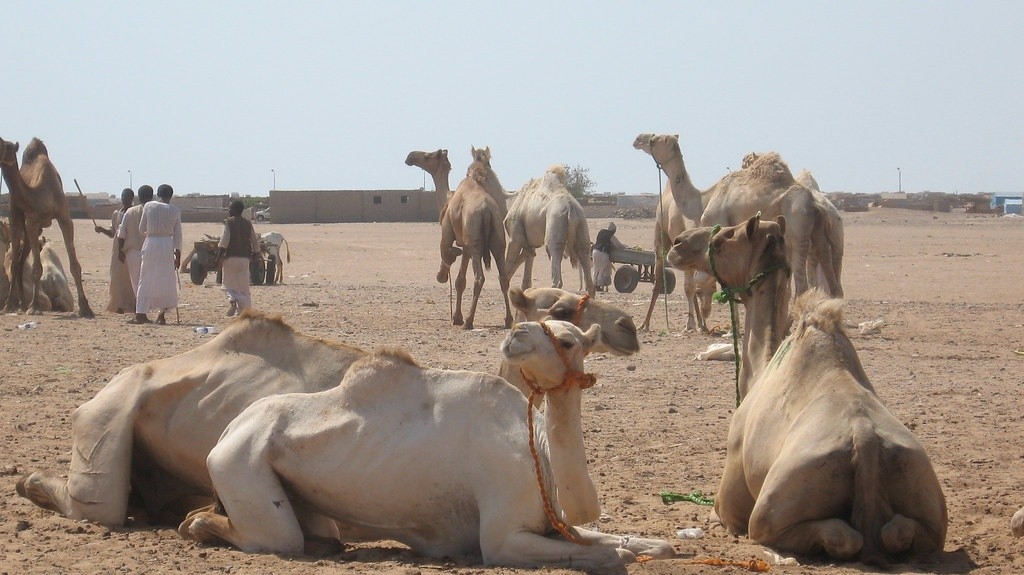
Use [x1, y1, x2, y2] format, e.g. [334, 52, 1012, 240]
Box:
[116, 184, 153, 297]
[127, 184, 183, 325]
[214, 200, 261, 317]
[95, 187, 137, 314]
[592, 221, 625, 293]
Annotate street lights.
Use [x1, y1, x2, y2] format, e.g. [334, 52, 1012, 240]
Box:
[271, 169, 275, 189]
[897, 167, 901, 193]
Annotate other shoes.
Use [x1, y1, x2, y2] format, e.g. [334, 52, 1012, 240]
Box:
[155, 317, 165, 325]
[227, 302, 236, 316]
[134, 313, 153, 324]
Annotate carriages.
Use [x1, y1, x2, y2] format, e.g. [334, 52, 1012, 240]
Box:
[563, 240, 677, 295]
[190, 231, 290, 286]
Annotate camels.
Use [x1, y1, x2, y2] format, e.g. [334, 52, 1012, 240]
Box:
[203, 231, 291, 285]
[1010, 507, 1024, 538]
[15, 280, 678, 574]
[632, 131, 949, 572]
[405, 144, 595, 330]
[0, 135, 96, 319]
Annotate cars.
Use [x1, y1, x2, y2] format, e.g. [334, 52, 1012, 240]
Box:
[254, 207, 270, 222]
